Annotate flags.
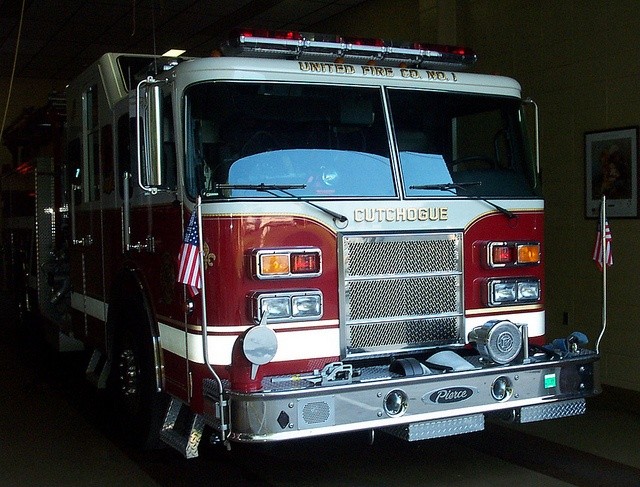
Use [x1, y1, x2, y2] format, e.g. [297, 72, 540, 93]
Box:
[592, 202, 615, 270]
[176, 207, 203, 298]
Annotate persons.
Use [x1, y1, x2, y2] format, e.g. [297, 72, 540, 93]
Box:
[596, 144, 631, 198]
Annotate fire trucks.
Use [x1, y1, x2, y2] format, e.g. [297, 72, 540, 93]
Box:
[0, 29, 613, 458]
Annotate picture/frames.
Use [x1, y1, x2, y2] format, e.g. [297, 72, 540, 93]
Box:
[584, 127, 637, 219]
